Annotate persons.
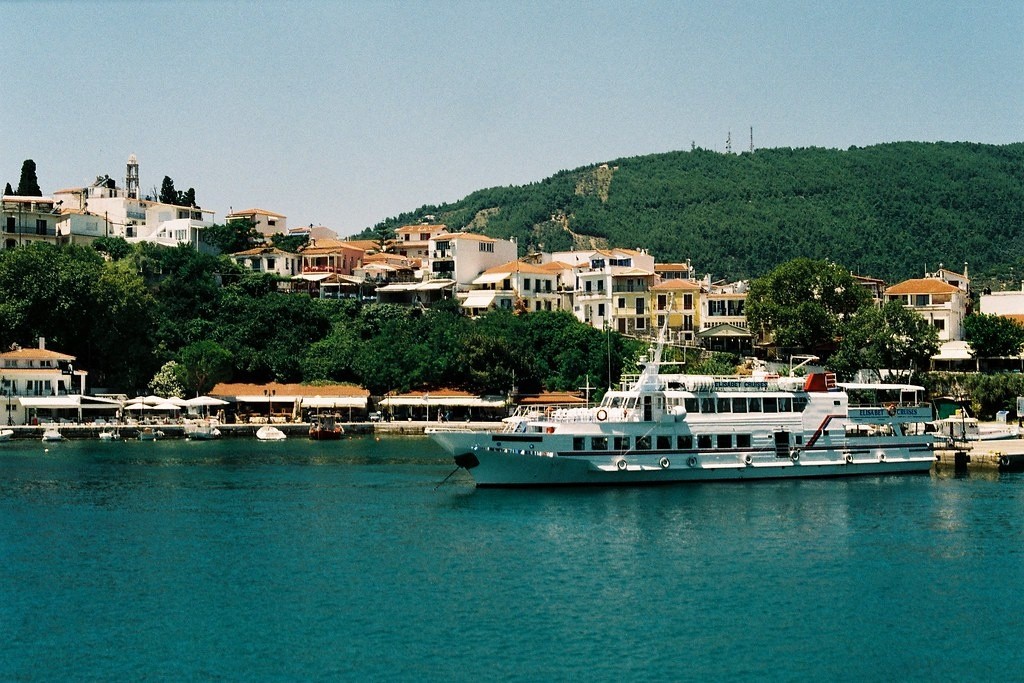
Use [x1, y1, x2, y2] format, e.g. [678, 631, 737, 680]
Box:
[382, 406, 391, 423]
[480, 407, 486, 422]
[216, 409, 224, 424]
[116, 408, 122, 425]
[437, 406, 450, 424]
[465, 409, 471, 423]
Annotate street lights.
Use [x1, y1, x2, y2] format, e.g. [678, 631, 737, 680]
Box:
[423, 382, 434, 422]
[136, 388, 149, 424]
[264, 388, 276, 424]
[500, 388, 512, 417]
[5, 389, 17, 426]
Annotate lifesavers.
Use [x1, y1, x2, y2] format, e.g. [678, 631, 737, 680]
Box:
[659, 457, 670, 469]
[743, 455, 753, 465]
[545, 408, 554, 417]
[877, 452, 887, 462]
[789, 451, 799, 462]
[845, 453, 854, 463]
[596, 410, 607, 421]
[618, 460, 627, 470]
[686, 456, 697, 467]
[887, 405, 896, 416]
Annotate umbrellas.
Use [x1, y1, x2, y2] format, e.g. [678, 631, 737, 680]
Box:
[124, 396, 230, 418]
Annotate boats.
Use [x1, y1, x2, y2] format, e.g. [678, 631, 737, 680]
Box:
[137, 427, 166, 442]
[834, 380, 934, 426]
[0, 429, 14, 441]
[255, 424, 287, 442]
[182, 418, 222, 440]
[306, 414, 345, 441]
[904, 395, 1024, 468]
[42, 425, 64, 441]
[423, 290, 942, 489]
[98, 427, 121, 441]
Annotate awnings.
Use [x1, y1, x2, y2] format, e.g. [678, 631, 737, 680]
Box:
[236, 396, 297, 402]
[19, 398, 80, 408]
[378, 398, 505, 408]
[301, 398, 368, 408]
